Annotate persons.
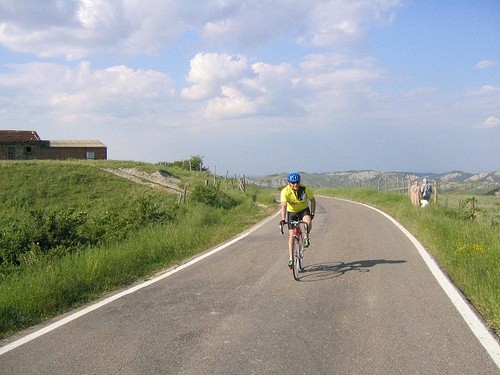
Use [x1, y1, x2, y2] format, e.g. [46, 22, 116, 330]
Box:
[279, 173, 316, 268]
[410, 178, 432, 209]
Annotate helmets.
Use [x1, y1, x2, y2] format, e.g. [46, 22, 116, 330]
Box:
[287, 173, 300, 183]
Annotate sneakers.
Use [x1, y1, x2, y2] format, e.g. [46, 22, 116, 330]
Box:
[288, 260, 294, 267]
[304, 239, 309, 246]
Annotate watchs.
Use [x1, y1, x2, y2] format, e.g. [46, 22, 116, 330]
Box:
[310, 212, 315, 216]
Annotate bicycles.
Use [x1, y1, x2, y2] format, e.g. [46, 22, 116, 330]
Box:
[279, 214, 309, 281]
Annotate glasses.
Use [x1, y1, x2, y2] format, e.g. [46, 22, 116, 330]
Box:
[291, 182, 299, 184]
